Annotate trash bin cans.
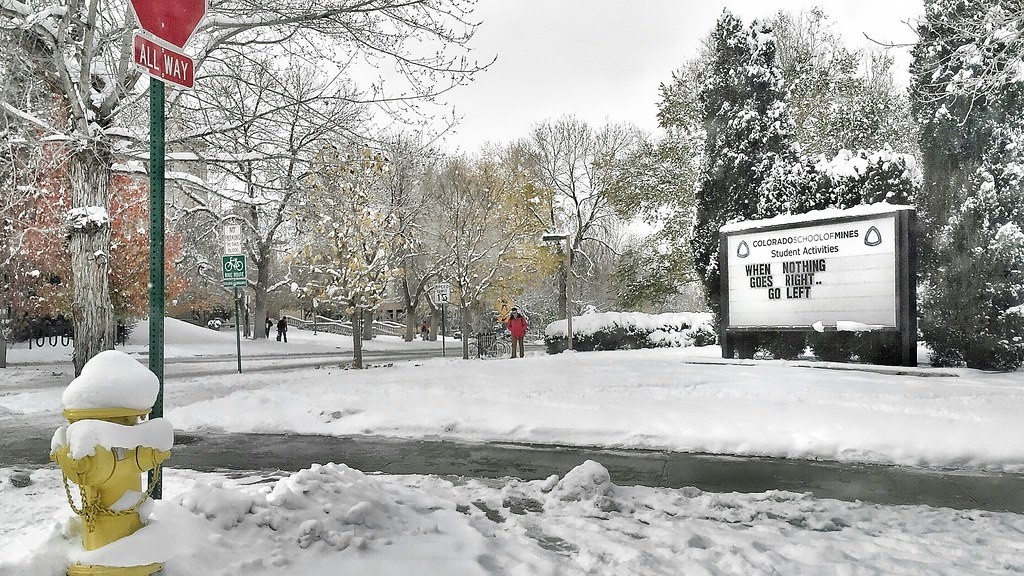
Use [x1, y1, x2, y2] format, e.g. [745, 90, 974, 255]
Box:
[214, 318, 221, 331]
[477, 332, 499, 360]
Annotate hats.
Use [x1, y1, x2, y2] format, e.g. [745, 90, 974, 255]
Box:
[283, 316, 287, 319]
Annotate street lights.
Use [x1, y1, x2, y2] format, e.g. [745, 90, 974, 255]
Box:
[542, 234, 573, 350]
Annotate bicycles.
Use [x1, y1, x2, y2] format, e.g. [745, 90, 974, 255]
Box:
[467, 336, 511, 359]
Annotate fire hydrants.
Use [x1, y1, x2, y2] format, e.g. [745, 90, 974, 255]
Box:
[52, 407, 171, 576]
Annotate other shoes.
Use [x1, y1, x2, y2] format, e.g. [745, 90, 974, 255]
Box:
[510, 357, 515, 359]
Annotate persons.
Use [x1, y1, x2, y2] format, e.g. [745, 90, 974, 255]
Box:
[265, 316, 273, 338]
[507, 308, 527, 359]
[421, 321, 429, 341]
[277, 316, 287, 343]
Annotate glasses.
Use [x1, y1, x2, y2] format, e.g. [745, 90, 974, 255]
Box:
[512, 312, 517, 314]
